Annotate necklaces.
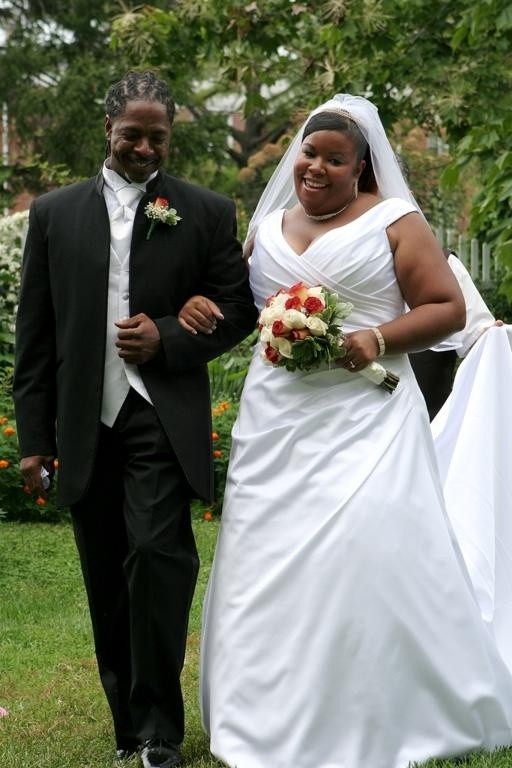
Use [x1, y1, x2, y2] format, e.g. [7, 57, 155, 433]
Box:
[301, 193, 356, 223]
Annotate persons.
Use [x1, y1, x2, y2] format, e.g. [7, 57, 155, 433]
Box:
[9, 70, 262, 766]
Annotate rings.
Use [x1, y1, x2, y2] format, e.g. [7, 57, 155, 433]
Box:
[348, 359, 357, 371]
[177, 89, 511, 766]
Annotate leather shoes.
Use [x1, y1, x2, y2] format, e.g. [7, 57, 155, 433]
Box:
[116, 739, 183, 768]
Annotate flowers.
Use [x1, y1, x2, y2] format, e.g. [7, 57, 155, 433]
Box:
[253, 280, 403, 397]
[144, 196, 184, 241]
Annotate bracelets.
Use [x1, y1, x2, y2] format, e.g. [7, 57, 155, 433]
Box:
[369, 325, 387, 359]
